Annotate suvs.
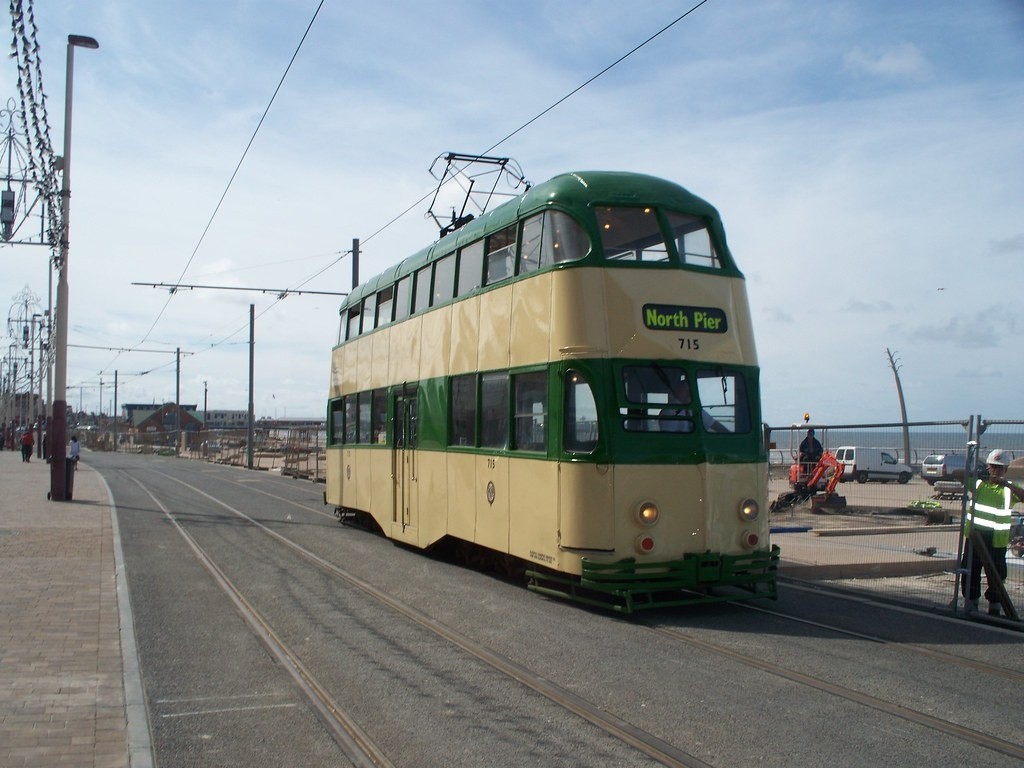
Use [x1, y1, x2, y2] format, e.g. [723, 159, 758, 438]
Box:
[922, 454, 990, 485]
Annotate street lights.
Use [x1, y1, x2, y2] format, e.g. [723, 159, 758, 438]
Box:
[49, 32, 102, 503]
[4, 345, 17, 449]
[28, 313, 42, 435]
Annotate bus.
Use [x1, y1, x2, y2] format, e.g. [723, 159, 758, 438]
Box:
[322, 151, 780, 618]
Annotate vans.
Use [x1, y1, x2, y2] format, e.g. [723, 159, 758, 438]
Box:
[835, 446, 913, 484]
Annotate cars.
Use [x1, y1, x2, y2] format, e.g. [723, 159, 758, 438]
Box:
[15, 422, 46, 433]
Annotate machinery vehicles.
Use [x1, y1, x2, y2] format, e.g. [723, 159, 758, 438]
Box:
[788, 413, 847, 514]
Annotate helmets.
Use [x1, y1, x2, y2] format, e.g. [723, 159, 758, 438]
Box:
[986, 449, 1010, 466]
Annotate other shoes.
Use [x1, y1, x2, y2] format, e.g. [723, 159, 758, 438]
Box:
[988, 606, 1001, 617]
[965, 600, 978, 610]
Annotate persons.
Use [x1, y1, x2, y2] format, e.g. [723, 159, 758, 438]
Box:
[797, 429, 823, 475]
[42, 433, 48, 459]
[656, 380, 732, 433]
[952, 450, 1024, 616]
[19, 427, 35, 463]
[0, 427, 16, 451]
[68, 435, 80, 470]
[29, 424, 34, 433]
